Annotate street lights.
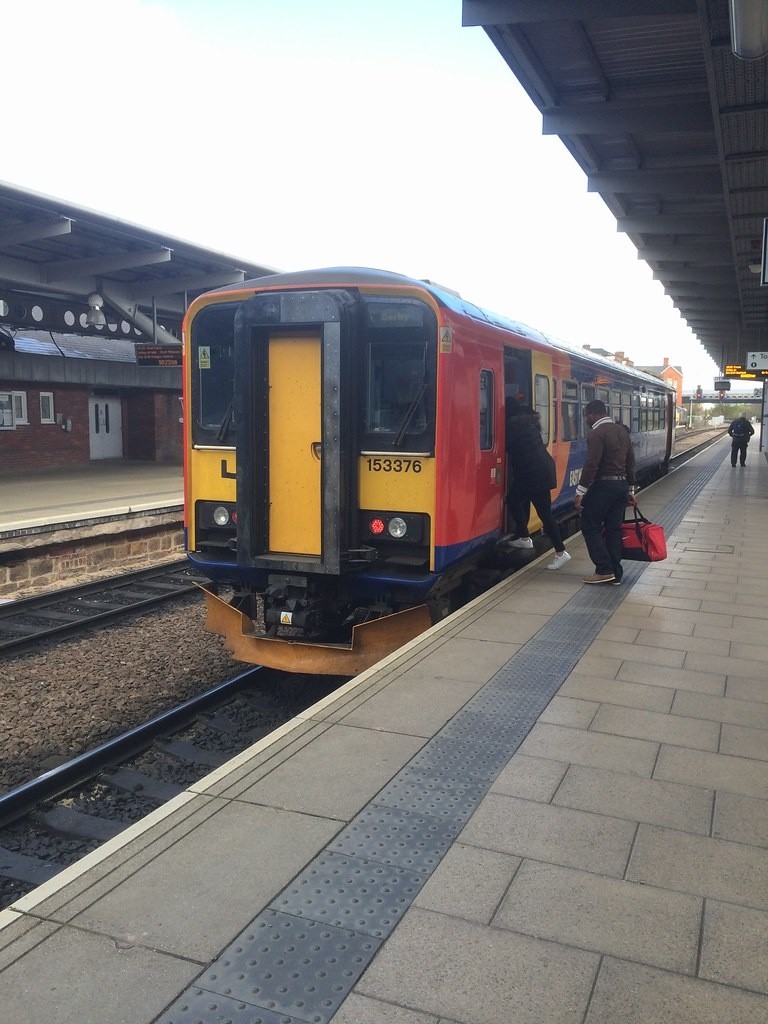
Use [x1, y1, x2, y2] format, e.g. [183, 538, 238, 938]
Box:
[689, 396, 693, 428]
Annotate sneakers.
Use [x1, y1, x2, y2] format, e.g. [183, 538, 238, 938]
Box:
[508, 538, 533, 548]
[547, 550, 571, 570]
[583, 573, 621, 585]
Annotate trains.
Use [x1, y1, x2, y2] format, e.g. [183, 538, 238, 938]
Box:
[638, 399, 688, 428]
[183, 266, 677, 675]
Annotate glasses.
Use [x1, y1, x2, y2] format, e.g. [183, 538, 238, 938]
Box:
[584, 411, 592, 419]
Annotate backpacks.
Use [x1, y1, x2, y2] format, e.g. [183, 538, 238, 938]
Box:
[732, 420, 746, 437]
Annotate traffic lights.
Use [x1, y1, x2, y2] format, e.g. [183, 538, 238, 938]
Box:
[720, 390, 724, 398]
[696, 389, 702, 399]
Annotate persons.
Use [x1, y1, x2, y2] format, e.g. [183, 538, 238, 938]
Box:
[505, 395, 571, 570]
[574, 400, 638, 585]
[728, 411, 755, 467]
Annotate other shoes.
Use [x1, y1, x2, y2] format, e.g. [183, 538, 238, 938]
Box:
[732, 464, 736, 467]
[741, 463, 746, 467]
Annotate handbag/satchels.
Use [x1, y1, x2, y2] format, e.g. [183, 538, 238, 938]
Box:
[602, 503, 667, 562]
